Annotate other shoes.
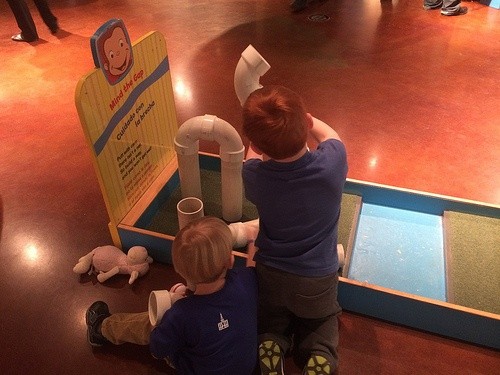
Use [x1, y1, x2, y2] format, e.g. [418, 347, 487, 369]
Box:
[257, 340, 285, 375]
[302, 351, 335, 375]
[422, 0, 468, 15]
[50, 23, 58, 33]
[289, 0, 308, 12]
[11, 34, 27, 41]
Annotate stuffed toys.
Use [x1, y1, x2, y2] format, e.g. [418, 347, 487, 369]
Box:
[73, 245, 153, 283]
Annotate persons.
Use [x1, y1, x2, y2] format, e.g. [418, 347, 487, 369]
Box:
[7, 0, 57, 41]
[240, 85, 348, 375]
[85, 216, 261, 375]
[424, 0, 467, 16]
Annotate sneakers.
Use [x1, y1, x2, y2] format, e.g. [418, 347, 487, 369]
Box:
[85, 300, 112, 350]
